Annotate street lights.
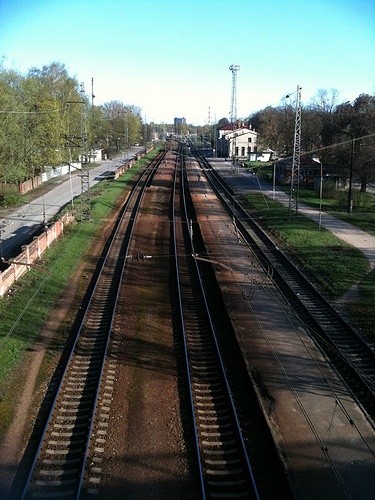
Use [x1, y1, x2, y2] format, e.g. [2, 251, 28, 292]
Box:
[313, 156, 323, 230]
